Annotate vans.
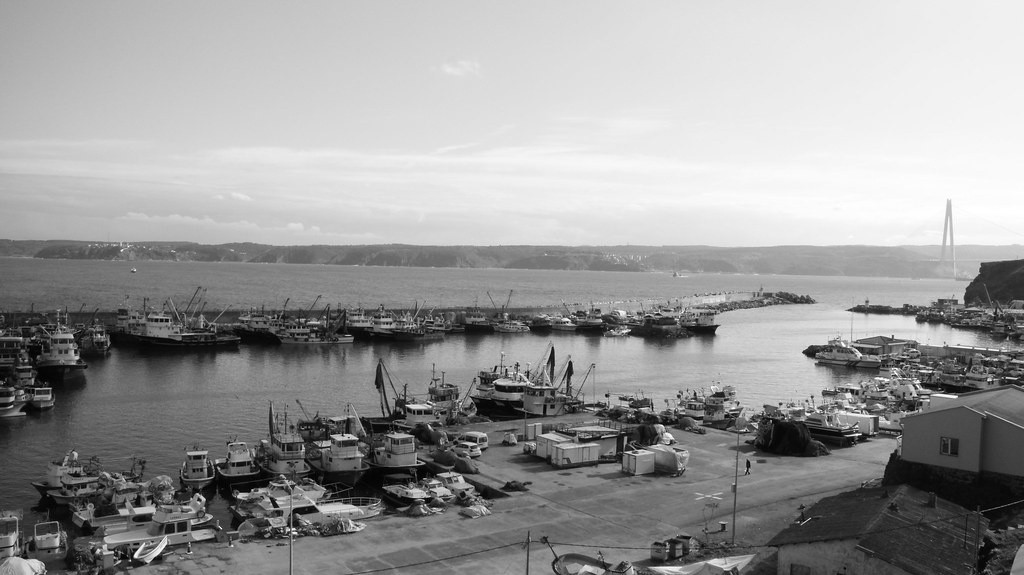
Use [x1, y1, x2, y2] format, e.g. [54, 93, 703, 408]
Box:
[459, 431, 489, 451]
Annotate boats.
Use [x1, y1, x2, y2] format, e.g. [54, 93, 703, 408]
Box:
[426, 311, 486, 333]
[491, 374, 528, 412]
[678, 308, 720, 336]
[494, 321, 529, 332]
[24, 386, 55, 408]
[419, 478, 456, 503]
[275, 330, 353, 343]
[660, 386, 744, 429]
[550, 317, 578, 330]
[0, 380, 27, 415]
[254, 400, 311, 482]
[30, 457, 145, 506]
[0, 311, 71, 373]
[365, 312, 445, 340]
[181, 451, 215, 490]
[602, 326, 632, 336]
[236, 311, 321, 333]
[214, 441, 260, 495]
[130, 268, 137, 272]
[611, 311, 681, 339]
[231, 478, 382, 521]
[899, 357, 1024, 392]
[911, 305, 1024, 340]
[322, 312, 375, 328]
[860, 377, 931, 410]
[821, 384, 861, 398]
[460, 323, 491, 331]
[0, 516, 25, 558]
[754, 400, 861, 445]
[115, 296, 239, 350]
[523, 315, 552, 331]
[46, 474, 172, 519]
[567, 312, 602, 325]
[467, 367, 516, 412]
[295, 364, 476, 440]
[26, 520, 68, 560]
[513, 384, 607, 417]
[381, 484, 431, 505]
[77, 317, 112, 358]
[69, 495, 187, 532]
[814, 345, 882, 367]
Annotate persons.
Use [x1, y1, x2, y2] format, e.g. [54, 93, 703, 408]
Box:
[744, 458, 752, 475]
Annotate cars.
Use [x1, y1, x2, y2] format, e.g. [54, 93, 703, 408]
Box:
[903, 349, 921, 359]
[450, 444, 481, 458]
